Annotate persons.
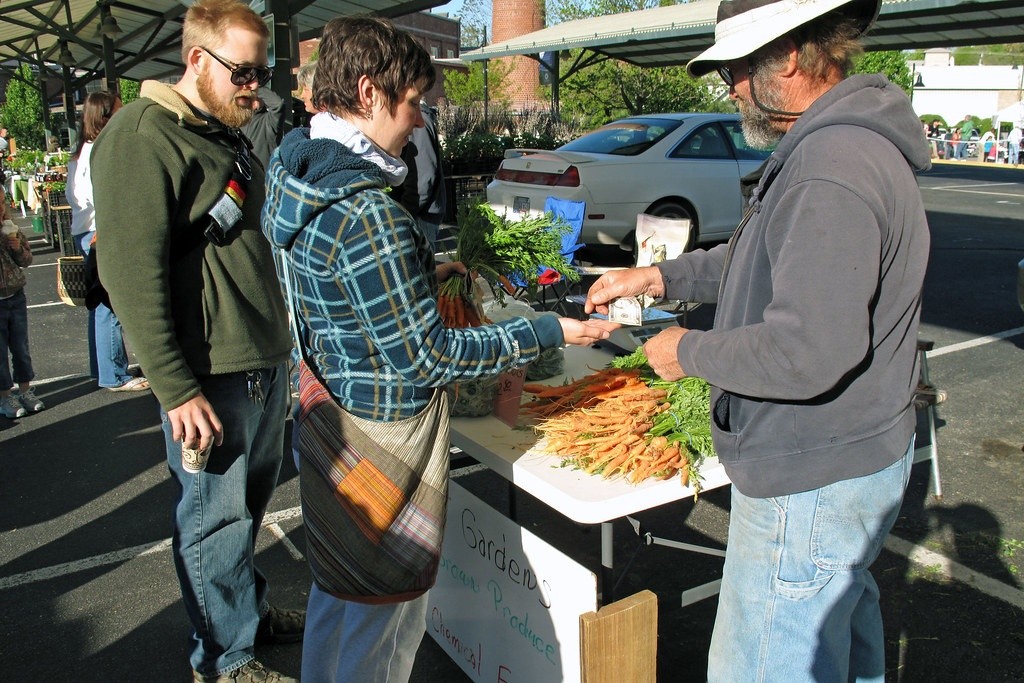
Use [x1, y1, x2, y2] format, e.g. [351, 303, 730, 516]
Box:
[586, 0, 930, 683]
[0, 184, 45, 416]
[388, 98, 446, 248]
[88, 0, 293, 683]
[298, 61, 328, 112]
[239, 87, 286, 170]
[257, 12, 622, 683]
[920, 115, 1024, 164]
[65, 90, 151, 390]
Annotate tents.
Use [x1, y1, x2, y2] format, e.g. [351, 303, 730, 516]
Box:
[992, 100, 1024, 163]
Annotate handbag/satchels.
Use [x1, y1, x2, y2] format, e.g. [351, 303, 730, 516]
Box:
[298, 358, 449, 602]
[57, 256, 86, 307]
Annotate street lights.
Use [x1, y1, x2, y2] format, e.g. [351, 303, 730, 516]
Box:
[911, 73, 927, 103]
[1011, 59, 1024, 102]
[475, 59, 490, 129]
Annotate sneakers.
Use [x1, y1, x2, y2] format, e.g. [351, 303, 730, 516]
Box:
[17, 390, 45, 411]
[0, 393, 27, 418]
[192, 659, 301, 683]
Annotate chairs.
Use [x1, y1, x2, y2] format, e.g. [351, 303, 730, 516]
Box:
[700, 136, 730, 158]
[565, 214, 702, 329]
[496, 195, 589, 317]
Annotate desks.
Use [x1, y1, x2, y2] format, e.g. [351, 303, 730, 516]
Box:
[12, 181, 28, 219]
[51, 205, 81, 256]
[450, 345, 947, 617]
[28, 177, 42, 215]
[43, 182, 78, 246]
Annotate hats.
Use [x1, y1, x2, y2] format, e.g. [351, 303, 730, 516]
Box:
[686, 0, 883, 77]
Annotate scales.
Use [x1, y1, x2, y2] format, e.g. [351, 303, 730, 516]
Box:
[589, 307, 683, 358]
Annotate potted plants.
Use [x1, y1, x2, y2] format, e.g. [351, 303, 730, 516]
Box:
[6, 144, 70, 181]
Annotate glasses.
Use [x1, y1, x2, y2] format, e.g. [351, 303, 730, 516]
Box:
[716, 60, 734, 86]
[200, 45, 274, 89]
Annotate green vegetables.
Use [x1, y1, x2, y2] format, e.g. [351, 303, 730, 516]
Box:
[5, 150, 69, 192]
[444, 349, 564, 419]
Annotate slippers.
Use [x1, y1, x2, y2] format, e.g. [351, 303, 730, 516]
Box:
[108, 377, 151, 392]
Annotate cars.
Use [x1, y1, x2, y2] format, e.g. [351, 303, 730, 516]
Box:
[931, 128, 1024, 163]
[487, 112, 779, 263]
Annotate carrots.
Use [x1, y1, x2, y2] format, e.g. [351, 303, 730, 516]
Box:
[436, 274, 515, 329]
[519, 365, 690, 486]
